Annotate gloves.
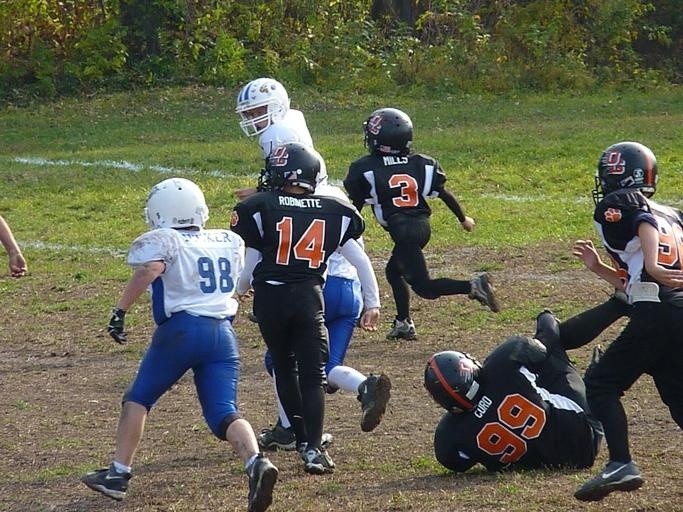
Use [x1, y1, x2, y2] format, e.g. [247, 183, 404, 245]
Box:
[106, 307, 128, 345]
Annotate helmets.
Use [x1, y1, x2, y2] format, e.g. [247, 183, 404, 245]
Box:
[591, 142, 659, 204]
[233, 78, 290, 137]
[146, 176, 210, 233]
[422, 351, 482, 416]
[360, 107, 414, 155]
[258, 142, 320, 194]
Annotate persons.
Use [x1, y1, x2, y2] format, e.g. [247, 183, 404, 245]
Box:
[573, 141, 683, 502]
[237, 147, 392, 452]
[343, 107, 500, 342]
[236, 78, 328, 182]
[81, 175, 278, 511]
[231, 143, 365, 474]
[0, 214, 27, 278]
[424, 278, 631, 472]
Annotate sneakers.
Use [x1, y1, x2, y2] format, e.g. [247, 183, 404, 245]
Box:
[591, 344, 604, 373]
[303, 449, 337, 476]
[242, 454, 278, 508]
[470, 274, 500, 314]
[575, 462, 642, 501]
[356, 375, 390, 432]
[385, 318, 417, 340]
[256, 416, 294, 452]
[80, 464, 132, 500]
